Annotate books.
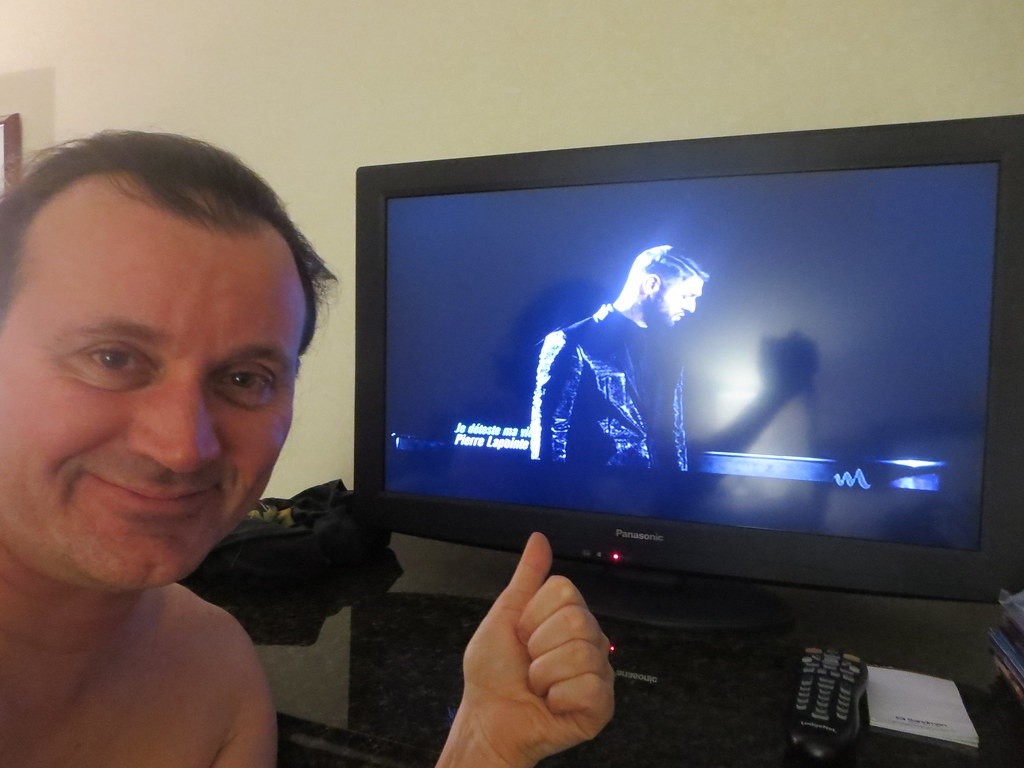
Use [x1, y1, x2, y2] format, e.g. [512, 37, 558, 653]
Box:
[985, 588, 1024, 707]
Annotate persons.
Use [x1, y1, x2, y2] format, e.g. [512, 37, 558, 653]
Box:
[530, 244, 709, 471]
[0, 132, 619, 768]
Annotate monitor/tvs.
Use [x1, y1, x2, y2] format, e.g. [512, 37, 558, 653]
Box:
[353, 114, 1024, 630]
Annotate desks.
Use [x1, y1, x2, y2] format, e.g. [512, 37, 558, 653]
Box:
[254, 536, 1024, 768]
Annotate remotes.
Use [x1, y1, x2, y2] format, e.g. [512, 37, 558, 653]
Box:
[789, 645, 869, 760]
